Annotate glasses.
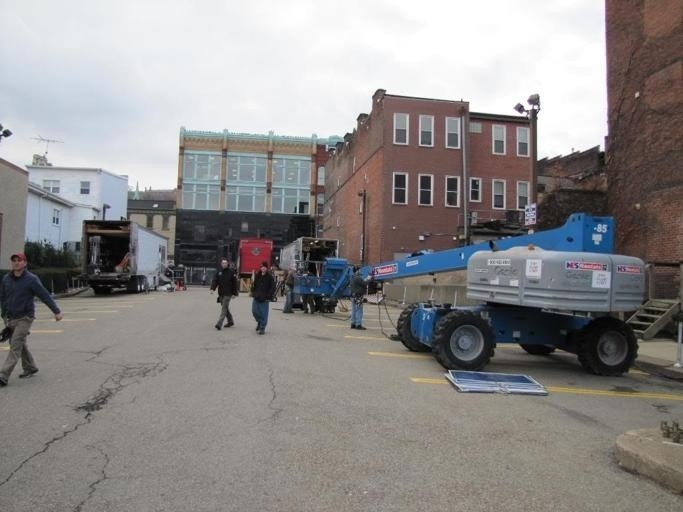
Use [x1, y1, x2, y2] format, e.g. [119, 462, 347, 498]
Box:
[12, 259, 23, 262]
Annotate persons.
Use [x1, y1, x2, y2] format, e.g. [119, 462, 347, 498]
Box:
[210, 257, 238, 330]
[0, 253, 63, 384]
[350, 266, 373, 330]
[250, 261, 277, 334]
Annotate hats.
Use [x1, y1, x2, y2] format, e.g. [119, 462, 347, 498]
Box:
[352, 265, 361, 272]
[260, 261, 268, 267]
[10, 252, 26, 261]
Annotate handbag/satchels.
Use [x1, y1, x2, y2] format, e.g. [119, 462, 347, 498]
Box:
[0, 327, 13, 343]
[217, 296, 221, 303]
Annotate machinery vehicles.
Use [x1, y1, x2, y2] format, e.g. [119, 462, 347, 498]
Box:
[284, 210, 648, 377]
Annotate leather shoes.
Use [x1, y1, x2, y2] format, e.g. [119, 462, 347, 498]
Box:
[19, 368, 39, 378]
[256, 326, 265, 335]
[215, 322, 234, 330]
[0, 377, 7, 386]
[351, 324, 367, 330]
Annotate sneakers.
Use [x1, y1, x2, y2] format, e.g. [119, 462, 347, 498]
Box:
[283, 311, 295, 313]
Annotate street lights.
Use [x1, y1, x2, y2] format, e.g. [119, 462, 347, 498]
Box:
[102, 203, 111, 220]
[0, 123, 12, 142]
[514, 91, 542, 233]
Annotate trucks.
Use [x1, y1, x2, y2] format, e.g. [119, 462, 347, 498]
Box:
[279, 236, 340, 286]
[81, 219, 169, 296]
